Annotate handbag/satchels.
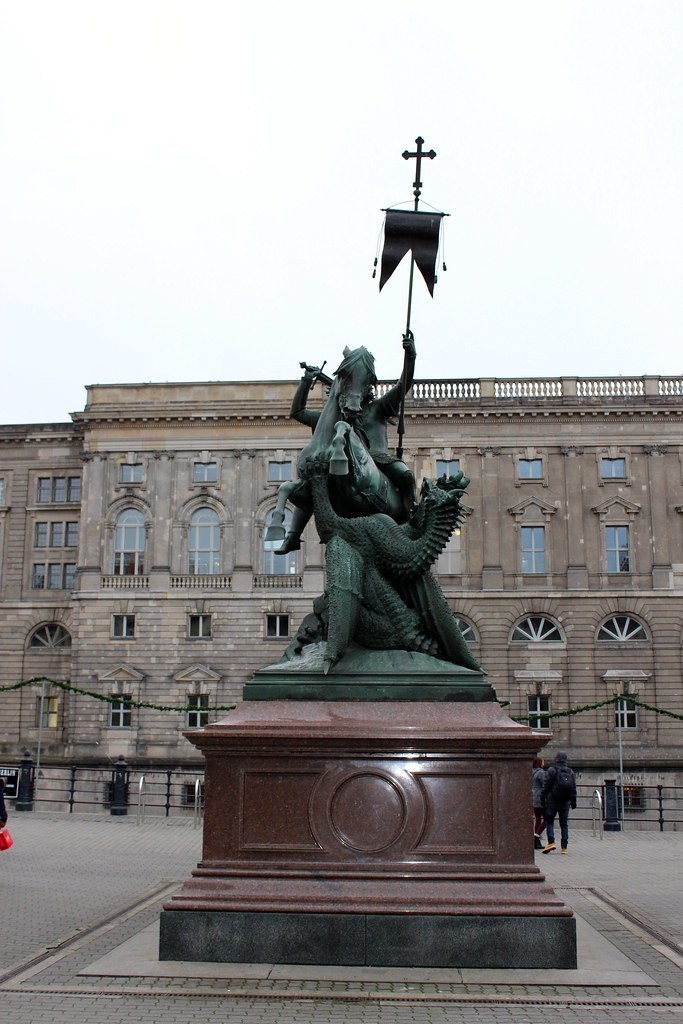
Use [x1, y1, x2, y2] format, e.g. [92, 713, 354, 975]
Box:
[0, 830, 13, 851]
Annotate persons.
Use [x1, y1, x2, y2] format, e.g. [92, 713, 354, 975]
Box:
[0, 783, 8, 829]
[531, 756, 547, 850]
[272, 328, 420, 555]
[541, 751, 577, 854]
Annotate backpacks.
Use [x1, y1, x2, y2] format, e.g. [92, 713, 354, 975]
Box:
[550, 766, 574, 802]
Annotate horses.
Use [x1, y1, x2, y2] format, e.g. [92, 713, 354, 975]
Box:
[261, 346, 408, 552]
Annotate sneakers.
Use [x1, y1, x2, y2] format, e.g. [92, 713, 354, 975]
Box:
[541, 843, 556, 854]
[561, 849, 567, 853]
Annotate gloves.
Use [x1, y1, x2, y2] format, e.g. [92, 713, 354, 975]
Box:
[569, 799, 576, 809]
[0, 822, 5, 828]
[540, 796, 546, 807]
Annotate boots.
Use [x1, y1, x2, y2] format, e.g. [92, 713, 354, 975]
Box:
[534, 834, 545, 849]
